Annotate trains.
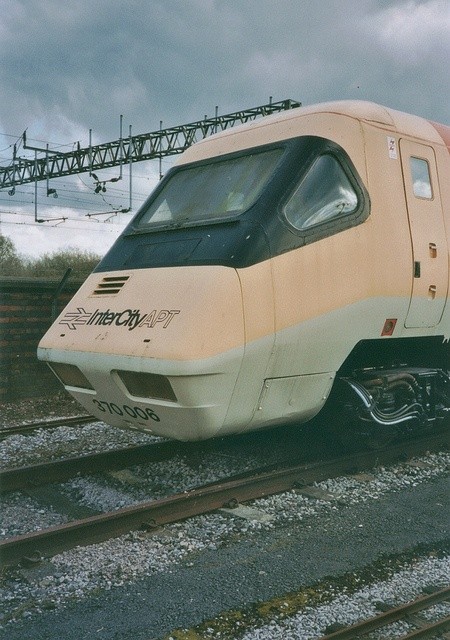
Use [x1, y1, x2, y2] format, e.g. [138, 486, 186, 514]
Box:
[38, 101, 450, 442]
